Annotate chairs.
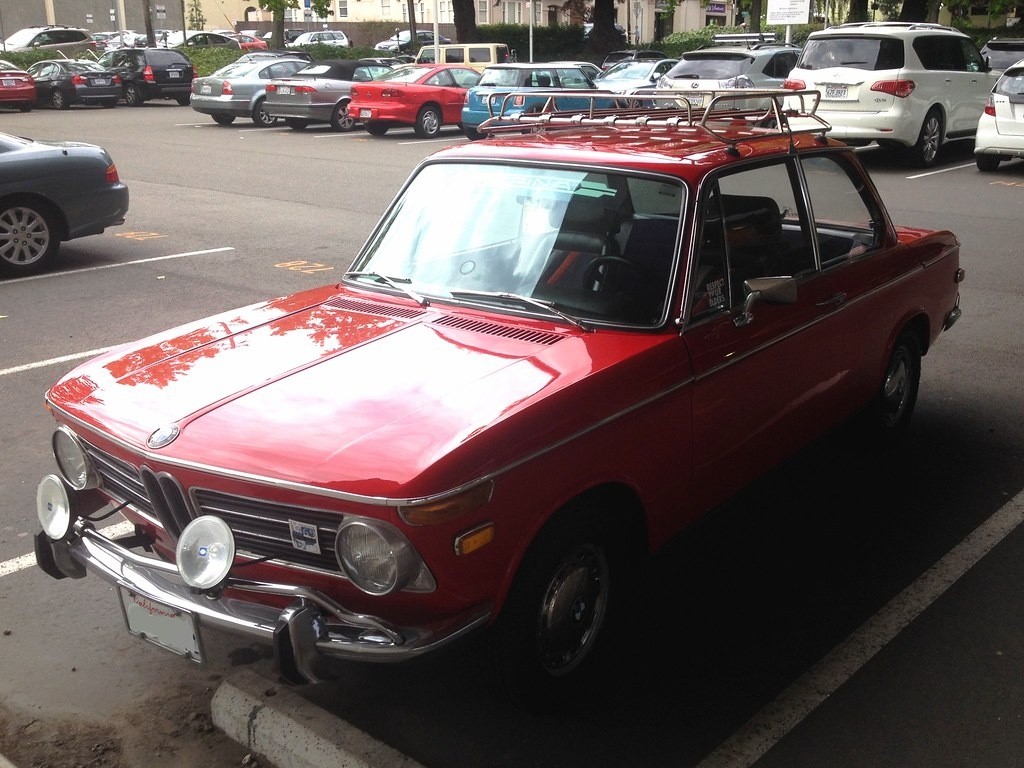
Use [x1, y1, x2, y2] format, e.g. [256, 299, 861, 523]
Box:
[634, 211, 727, 326]
[515, 197, 620, 316]
[710, 194, 785, 294]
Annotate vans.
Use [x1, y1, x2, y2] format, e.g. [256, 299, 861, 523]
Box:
[416, 43, 511, 77]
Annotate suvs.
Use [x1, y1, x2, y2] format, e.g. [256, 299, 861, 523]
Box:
[782, 21, 996, 169]
[293, 31, 349, 52]
[657, 43, 804, 112]
[35, 88, 966, 713]
[97, 48, 199, 105]
[0, 24, 97, 65]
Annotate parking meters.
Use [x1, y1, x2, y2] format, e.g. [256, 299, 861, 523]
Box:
[395, 28, 401, 53]
[162, 32, 168, 50]
[284, 28, 290, 47]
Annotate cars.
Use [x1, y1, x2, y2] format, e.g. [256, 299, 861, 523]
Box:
[602, 51, 669, 63]
[238, 51, 314, 63]
[190, 59, 310, 127]
[0, 60, 36, 111]
[979, 36, 1024, 80]
[599, 59, 680, 87]
[26, 59, 123, 110]
[91, 29, 305, 53]
[0, 131, 129, 276]
[262, 60, 395, 132]
[974, 58, 1024, 170]
[549, 61, 603, 83]
[462, 63, 641, 141]
[359, 55, 417, 67]
[376, 30, 452, 58]
[348, 63, 482, 139]
